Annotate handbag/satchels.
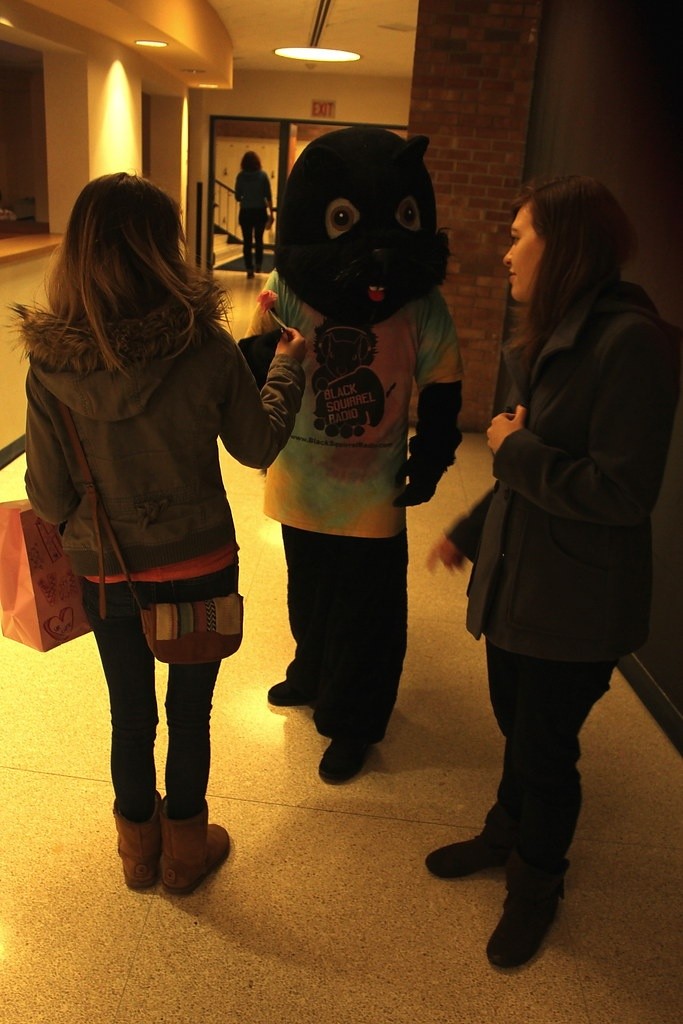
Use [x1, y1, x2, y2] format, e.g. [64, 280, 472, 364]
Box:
[0, 498, 94, 652]
[141, 593, 243, 665]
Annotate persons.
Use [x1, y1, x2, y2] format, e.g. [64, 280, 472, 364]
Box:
[234, 151, 274, 279]
[426, 174, 682, 970]
[25, 172, 305, 897]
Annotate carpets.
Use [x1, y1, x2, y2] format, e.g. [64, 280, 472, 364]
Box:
[213, 252, 279, 275]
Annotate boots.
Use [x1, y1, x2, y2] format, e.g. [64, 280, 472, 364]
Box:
[425, 802, 520, 878]
[485, 854, 570, 967]
[159, 795, 230, 895]
[113, 789, 163, 887]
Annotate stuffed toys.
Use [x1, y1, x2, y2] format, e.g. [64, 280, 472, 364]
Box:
[245, 126, 462, 785]
